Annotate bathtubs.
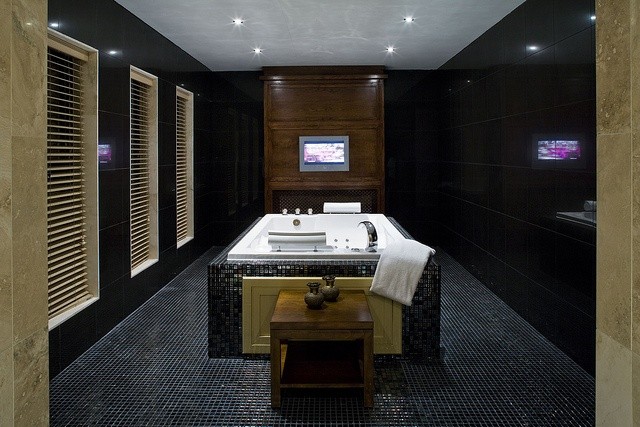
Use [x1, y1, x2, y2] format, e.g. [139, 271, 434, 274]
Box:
[228, 212, 406, 260]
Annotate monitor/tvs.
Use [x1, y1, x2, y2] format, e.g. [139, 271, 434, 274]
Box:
[303, 140, 345, 164]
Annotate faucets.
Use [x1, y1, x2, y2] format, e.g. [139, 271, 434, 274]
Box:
[357, 220, 379, 252]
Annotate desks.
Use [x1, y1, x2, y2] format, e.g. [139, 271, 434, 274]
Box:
[271, 289, 373, 408]
